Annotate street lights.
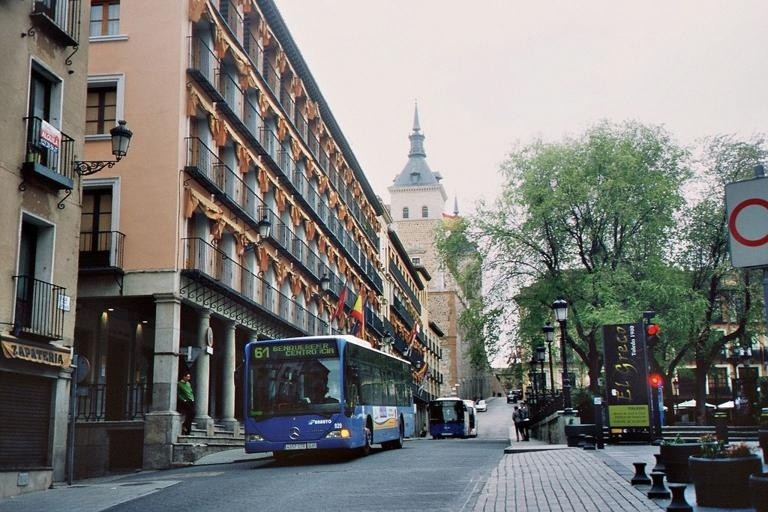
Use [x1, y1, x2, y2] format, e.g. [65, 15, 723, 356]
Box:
[552, 297, 572, 412]
[542, 321, 555, 398]
[522, 347, 546, 415]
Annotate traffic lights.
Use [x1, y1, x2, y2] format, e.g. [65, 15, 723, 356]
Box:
[648, 373, 664, 389]
[646, 324, 659, 347]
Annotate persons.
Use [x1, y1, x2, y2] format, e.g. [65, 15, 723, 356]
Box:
[518, 402, 530, 441]
[177, 372, 196, 435]
[512, 406, 525, 441]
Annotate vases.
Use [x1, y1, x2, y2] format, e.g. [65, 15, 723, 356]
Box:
[688, 454, 763, 509]
[758, 429, 768, 464]
[749, 472, 768, 512]
[565, 424, 596, 448]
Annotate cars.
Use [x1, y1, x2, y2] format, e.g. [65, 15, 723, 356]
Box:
[475, 399, 488, 412]
[507, 390, 520, 404]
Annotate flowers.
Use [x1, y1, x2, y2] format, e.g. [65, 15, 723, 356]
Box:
[697, 434, 761, 459]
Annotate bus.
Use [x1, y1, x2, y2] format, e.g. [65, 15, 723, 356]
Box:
[242, 334, 418, 461]
[428, 397, 478, 439]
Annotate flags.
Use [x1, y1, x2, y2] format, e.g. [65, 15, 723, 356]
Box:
[416, 362, 428, 380]
[405, 321, 417, 356]
[331, 280, 365, 339]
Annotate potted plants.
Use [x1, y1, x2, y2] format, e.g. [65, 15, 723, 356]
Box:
[659, 433, 703, 483]
